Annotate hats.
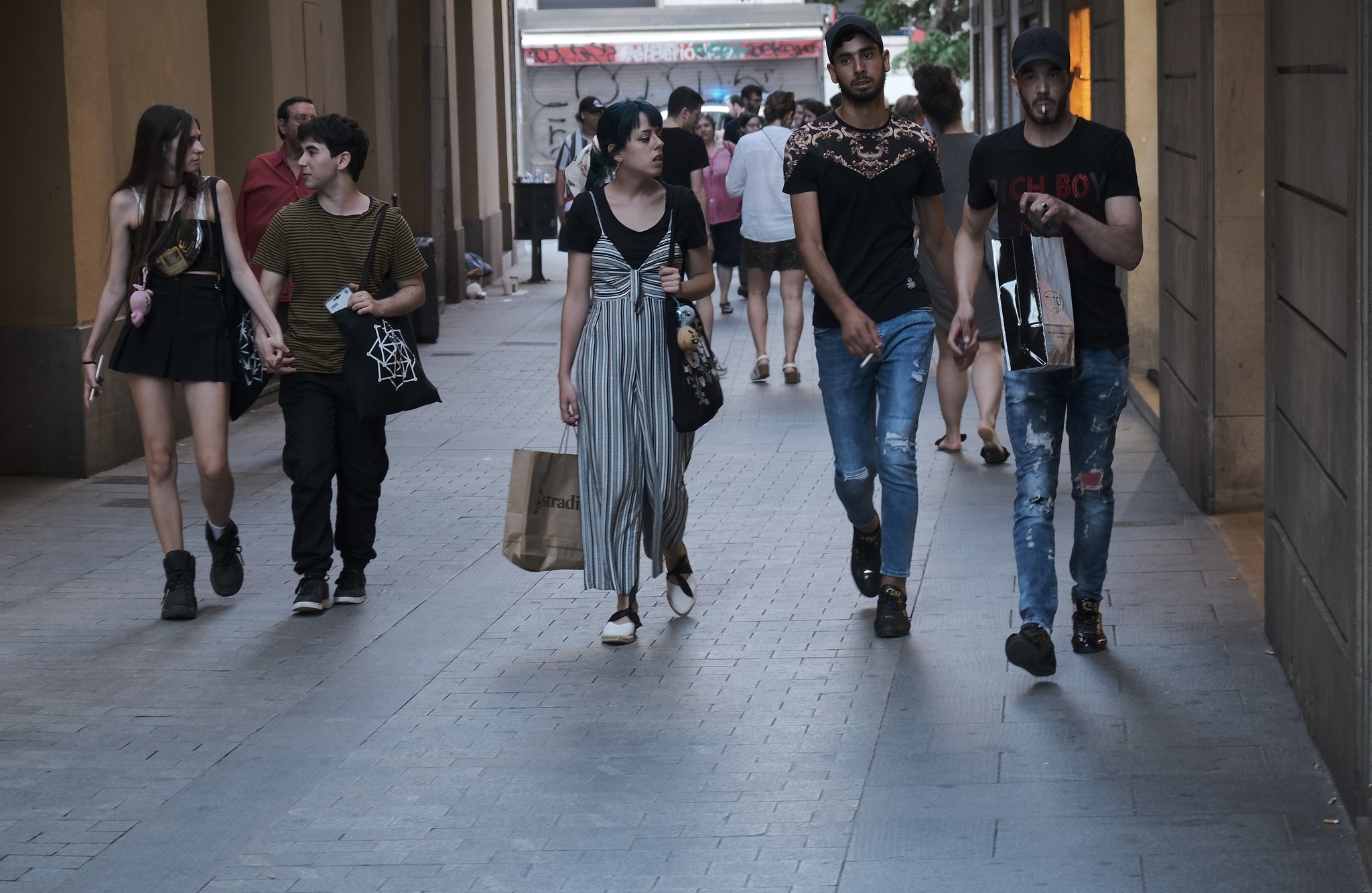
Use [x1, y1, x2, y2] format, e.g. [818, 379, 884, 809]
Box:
[824, 15, 883, 60]
[1012, 26, 1071, 77]
[579, 96, 606, 111]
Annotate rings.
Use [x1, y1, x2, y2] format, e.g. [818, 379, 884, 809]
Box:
[1041, 202, 1048, 211]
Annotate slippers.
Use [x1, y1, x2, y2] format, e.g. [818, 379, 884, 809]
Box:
[935, 433, 967, 454]
[981, 445, 1011, 464]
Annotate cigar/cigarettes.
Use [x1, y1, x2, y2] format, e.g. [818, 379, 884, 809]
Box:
[1042, 104, 1046, 114]
[859, 344, 882, 368]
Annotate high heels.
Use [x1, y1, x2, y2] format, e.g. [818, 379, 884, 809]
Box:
[750, 355, 770, 381]
[781, 362, 800, 384]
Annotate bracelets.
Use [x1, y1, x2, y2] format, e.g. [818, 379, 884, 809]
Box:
[82, 361, 97, 364]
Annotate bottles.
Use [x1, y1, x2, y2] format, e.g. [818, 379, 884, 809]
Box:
[533, 166, 541, 183]
[523, 170, 532, 183]
[543, 170, 550, 184]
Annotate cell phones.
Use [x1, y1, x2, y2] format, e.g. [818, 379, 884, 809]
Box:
[89, 354, 105, 401]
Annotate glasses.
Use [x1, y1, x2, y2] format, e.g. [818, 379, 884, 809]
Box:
[751, 98, 763, 102]
[745, 122, 763, 129]
[288, 113, 318, 124]
[589, 108, 602, 114]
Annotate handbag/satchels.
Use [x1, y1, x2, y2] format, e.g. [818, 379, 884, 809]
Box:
[219, 266, 272, 421]
[663, 292, 722, 434]
[143, 172, 203, 279]
[989, 210, 1076, 374]
[502, 413, 584, 573]
[329, 284, 443, 417]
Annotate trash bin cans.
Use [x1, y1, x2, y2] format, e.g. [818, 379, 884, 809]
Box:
[512, 176, 559, 240]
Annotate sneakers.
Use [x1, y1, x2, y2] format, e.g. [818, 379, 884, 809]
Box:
[851, 511, 882, 598]
[332, 570, 366, 604]
[1005, 621, 1056, 676]
[712, 356, 728, 376]
[1071, 597, 1109, 653]
[291, 573, 333, 612]
[160, 549, 197, 620]
[205, 516, 245, 597]
[873, 584, 911, 638]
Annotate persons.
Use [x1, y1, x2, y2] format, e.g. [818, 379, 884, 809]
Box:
[236, 96, 317, 324]
[551, 61, 1010, 466]
[252, 114, 425, 612]
[562, 101, 716, 646]
[81, 97, 288, 621]
[781, 18, 980, 637]
[948, 25, 1145, 677]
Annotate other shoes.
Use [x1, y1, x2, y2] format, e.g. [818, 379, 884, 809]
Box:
[601, 587, 643, 645]
[738, 285, 749, 298]
[720, 300, 733, 314]
[666, 542, 697, 617]
